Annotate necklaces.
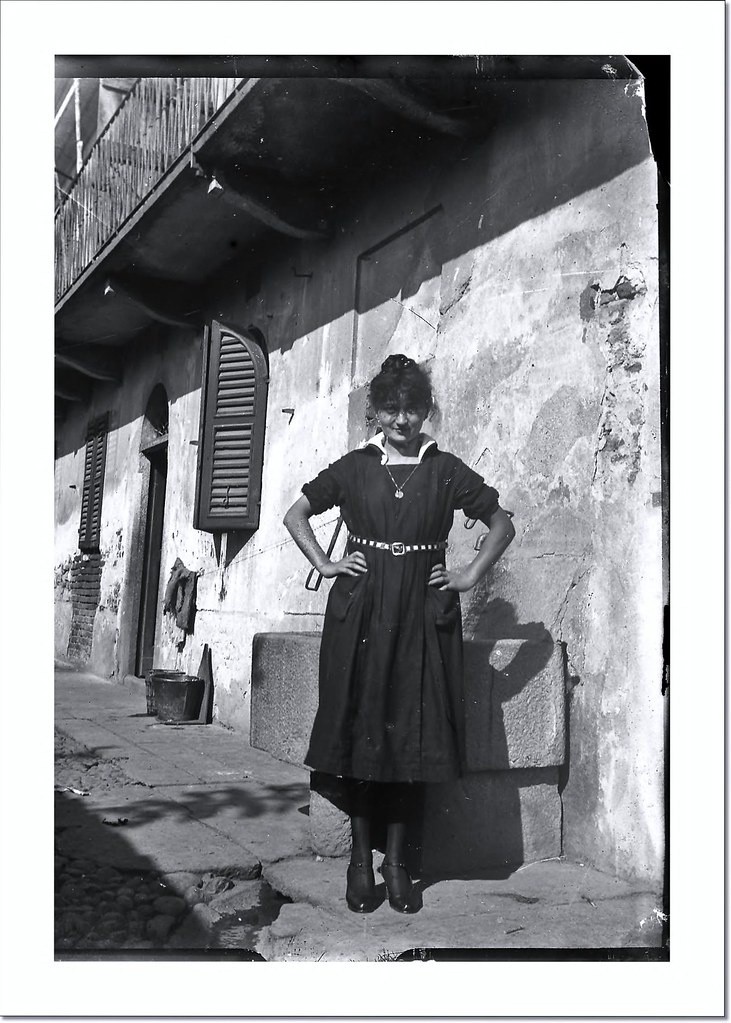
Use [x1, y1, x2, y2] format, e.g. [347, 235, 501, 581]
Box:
[385, 463, 419, 499]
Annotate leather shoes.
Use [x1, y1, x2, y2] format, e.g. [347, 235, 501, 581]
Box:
[381, 862, 423, 913]
[346, 863, 375, 913]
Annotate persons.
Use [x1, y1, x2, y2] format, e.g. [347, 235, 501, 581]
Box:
[283, 353, 515, 913]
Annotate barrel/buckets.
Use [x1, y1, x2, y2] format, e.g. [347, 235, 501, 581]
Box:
[152, 674, 204, 720]
